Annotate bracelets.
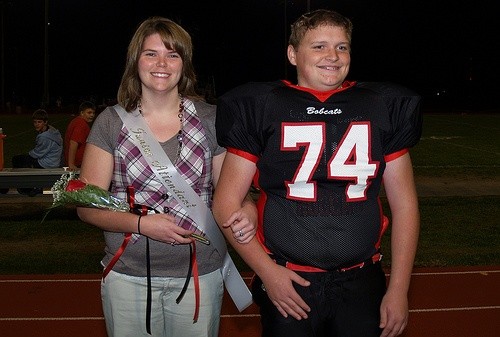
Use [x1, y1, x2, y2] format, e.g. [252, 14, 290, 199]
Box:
[137, 215, 143, 235]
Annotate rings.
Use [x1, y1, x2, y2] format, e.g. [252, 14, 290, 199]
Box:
[171, 239, 177, 246]
[239, 230, 244, 236]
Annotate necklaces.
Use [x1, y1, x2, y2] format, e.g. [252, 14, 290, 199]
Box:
[136, 92, 185, 167]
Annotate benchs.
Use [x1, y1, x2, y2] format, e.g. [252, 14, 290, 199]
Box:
[0, 168, 80, 176]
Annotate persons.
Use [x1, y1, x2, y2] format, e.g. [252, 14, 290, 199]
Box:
[211, 10, 420, 337]
[12, 109, 63, 195]
[65, 101, 96, 175]
[76, 18, 258, 337]
[5, 95, 66, 115]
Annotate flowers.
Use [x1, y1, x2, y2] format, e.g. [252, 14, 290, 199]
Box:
[39, 166, 210, 246]
